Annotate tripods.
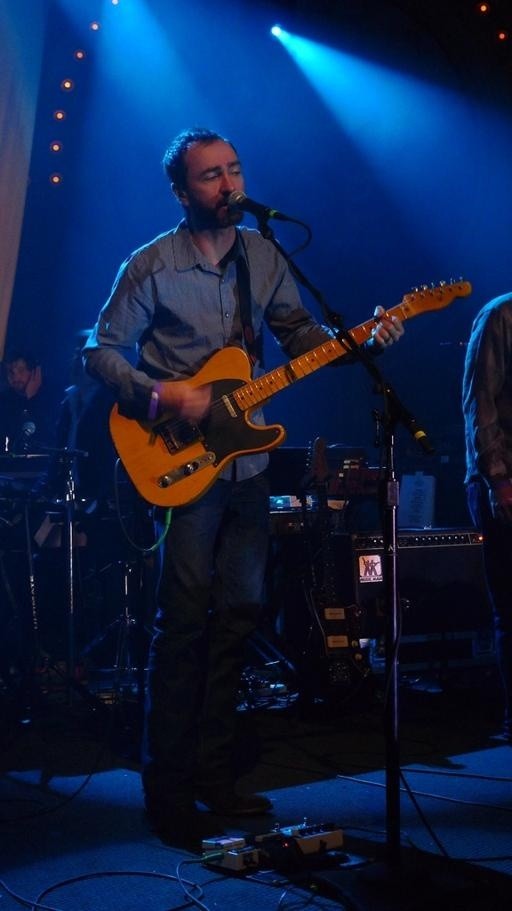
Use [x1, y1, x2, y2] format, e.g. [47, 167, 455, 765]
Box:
[0, 486, 152, 714]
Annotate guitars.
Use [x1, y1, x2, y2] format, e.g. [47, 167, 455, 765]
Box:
[110, 277, 472, 508]
[305, 438, 384, 709]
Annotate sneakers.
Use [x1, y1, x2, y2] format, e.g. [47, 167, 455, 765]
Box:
[150, 785, 273, 858]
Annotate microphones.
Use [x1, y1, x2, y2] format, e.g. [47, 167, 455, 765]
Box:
[228, 190, 286, 222]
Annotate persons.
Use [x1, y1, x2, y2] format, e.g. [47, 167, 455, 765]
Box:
[458, 290, 510, 753]
[79, 122, 412, 851]
[2, 296, 511, 758]
